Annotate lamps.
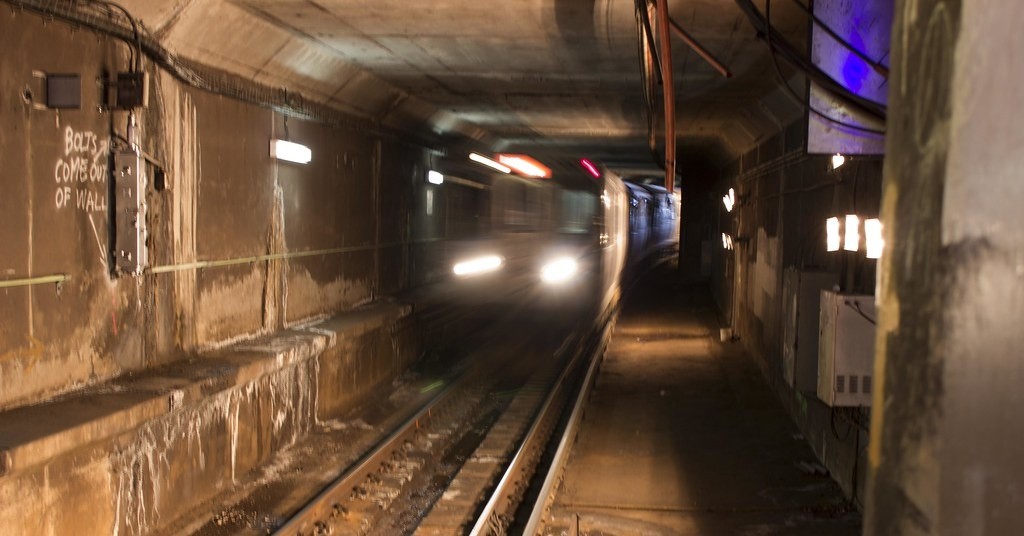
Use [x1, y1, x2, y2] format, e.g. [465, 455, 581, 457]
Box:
[269, 139, 311, 164]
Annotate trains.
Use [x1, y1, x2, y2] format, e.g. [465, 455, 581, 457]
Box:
[470, 155, 681, 319]
